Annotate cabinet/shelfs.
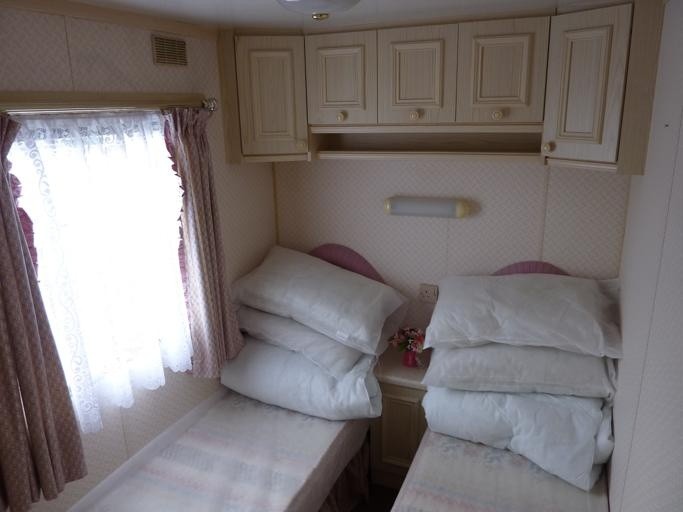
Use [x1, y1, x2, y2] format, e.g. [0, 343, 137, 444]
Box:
[539, 2, 666, 177]
[304, 15, 551, 165]
[216, 26, 316, 168]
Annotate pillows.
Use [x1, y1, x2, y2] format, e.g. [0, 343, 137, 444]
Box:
[230, 244, 411, 357]
[422, 346, 619, 403]
[421, 274, 623, 360]
[235, 305, 364, 382]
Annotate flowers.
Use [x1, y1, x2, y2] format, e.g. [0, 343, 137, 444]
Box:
[387, 325, 425, 353]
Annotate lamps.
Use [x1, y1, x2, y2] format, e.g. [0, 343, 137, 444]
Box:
[384, 195, 471, 219]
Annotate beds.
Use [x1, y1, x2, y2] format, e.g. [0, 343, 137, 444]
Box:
[64, 244, 392, 511]
[391, 258, 611, 512]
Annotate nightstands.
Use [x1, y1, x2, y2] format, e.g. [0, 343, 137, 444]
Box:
[369, 363, 429, 489]
[371, 348, 428, 471]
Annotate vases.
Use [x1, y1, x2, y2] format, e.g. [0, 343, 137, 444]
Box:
[402, 348, 418, 369]
